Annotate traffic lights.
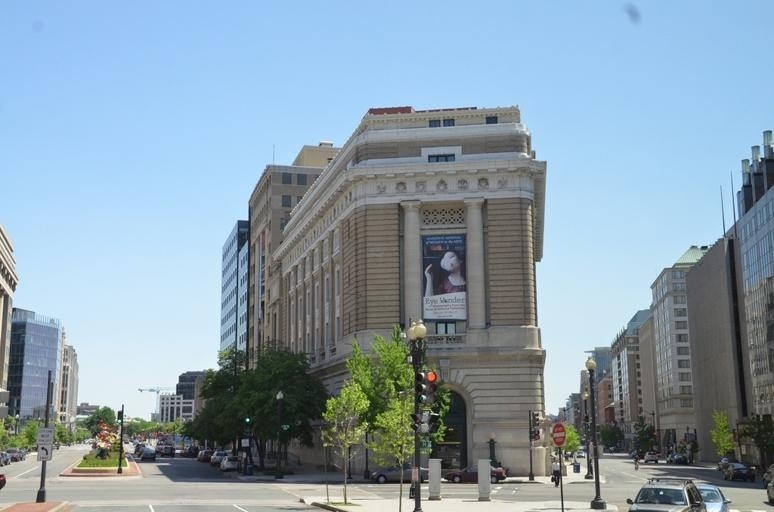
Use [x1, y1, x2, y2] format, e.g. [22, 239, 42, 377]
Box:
[409, 414, 441, 433]
[531, 411, 540, 440]
[117, 411, 122, 424]
[416, 371, 438, 405]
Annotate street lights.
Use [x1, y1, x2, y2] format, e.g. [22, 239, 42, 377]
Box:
[407, 319, 427, 512]
[14, 414, 20, 435]
[275, 391, 284, 479]
[581, 356, 607, 509]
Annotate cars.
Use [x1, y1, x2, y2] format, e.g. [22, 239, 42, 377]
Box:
[370, 462, 507, 484]
[0, 449, 26, 467]
[627, 477, 731, 512]
[124, 439, 242, 471]
[0, 474, 6, 490]
[666, 454, 687, 464]
[577, 451, 585, 458]
[762, 464, 774, 503]
[718, 458, 750, 481]
[644, 452, 658, 464]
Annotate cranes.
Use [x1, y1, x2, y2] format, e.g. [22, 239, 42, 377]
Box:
[137, 386, 172, 414]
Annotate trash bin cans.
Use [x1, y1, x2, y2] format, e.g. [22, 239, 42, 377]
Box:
[247, 465, 253, 474]
[573, 463, 580, 472]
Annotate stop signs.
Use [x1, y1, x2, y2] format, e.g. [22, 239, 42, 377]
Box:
[551, 424, 566, 446]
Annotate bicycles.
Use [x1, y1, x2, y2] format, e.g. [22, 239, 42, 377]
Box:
[633, 458, 640, 470]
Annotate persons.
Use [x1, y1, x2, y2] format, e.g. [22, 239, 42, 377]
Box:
[552, 459, 560, 487]
[634, 454, 640, 470]
[424, 250, 466, 296]
